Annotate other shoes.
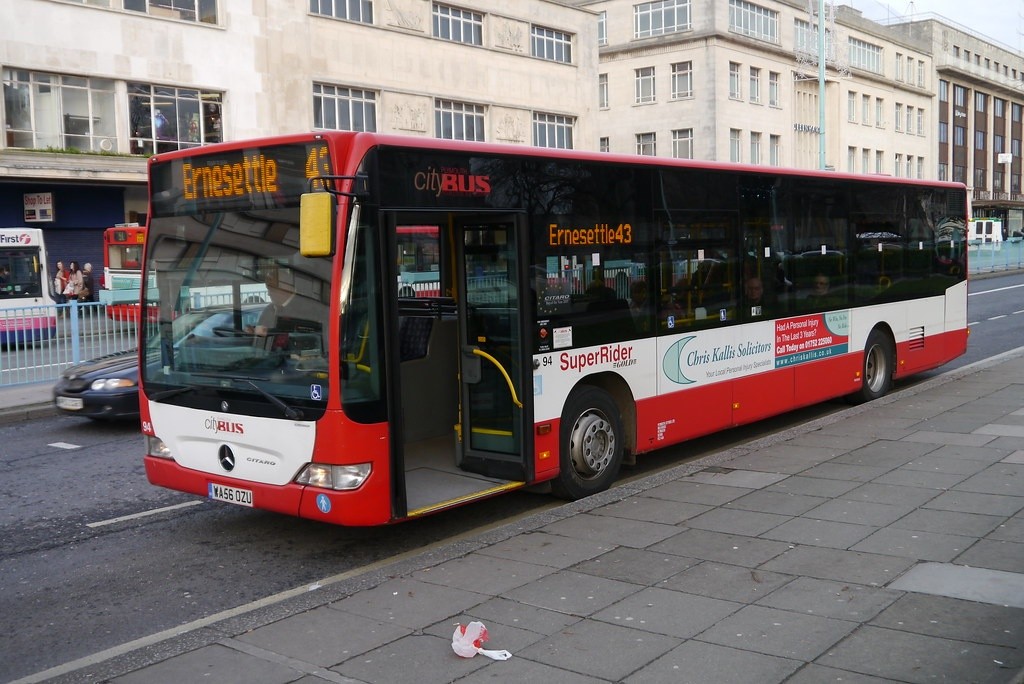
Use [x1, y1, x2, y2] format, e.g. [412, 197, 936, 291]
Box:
[57, 305, 100, 319]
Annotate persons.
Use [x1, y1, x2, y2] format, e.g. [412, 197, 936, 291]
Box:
[77, 262, 98, 316]
[54, 260, 69, 318]
[244, 268, 322, 369]
[572, 251, 851, 325]
[65, 261, 84, 318]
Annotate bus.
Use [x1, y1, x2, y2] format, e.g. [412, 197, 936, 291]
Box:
[100, 223, 441, 325]
[0, 228, 56, 344]
[137, 129, 971, 527]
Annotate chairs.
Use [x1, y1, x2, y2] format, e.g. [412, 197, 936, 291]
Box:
[546, 238, 952, 331]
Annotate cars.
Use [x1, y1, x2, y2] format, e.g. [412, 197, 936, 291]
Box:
[52, 303, 270, 418]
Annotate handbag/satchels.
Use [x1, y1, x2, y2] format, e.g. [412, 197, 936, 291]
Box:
[62, 280, 75, 295]
[79, 288, 89, 300]
